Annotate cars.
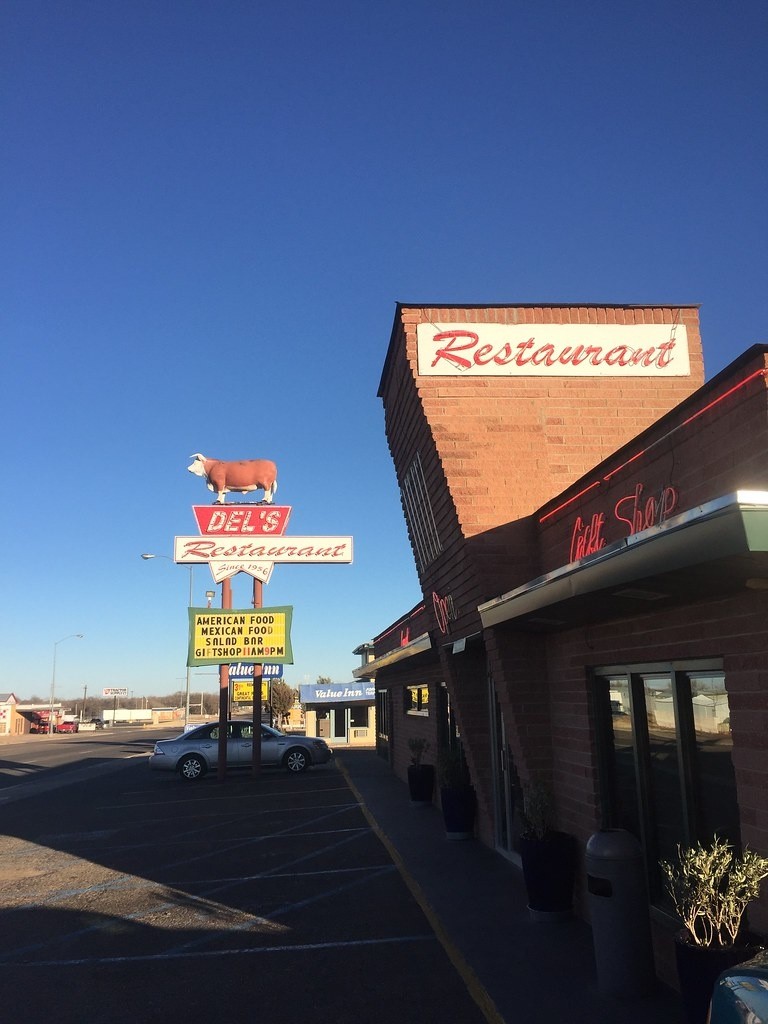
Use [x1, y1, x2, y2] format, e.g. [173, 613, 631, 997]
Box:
[147, 719, 334, 782]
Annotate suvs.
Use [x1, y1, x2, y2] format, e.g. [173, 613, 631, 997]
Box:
[39, 721, 56, 734]
[90, 718, 104, 729]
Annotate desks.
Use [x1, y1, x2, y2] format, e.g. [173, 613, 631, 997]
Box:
[706, 949, 768, 1024]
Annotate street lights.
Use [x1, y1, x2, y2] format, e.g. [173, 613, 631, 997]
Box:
[50, 634, 83, 734]
[141, 553, 192, 724]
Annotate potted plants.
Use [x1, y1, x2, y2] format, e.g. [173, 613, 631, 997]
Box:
[658, 833, 768, 1024]
[406, 735, 434, 807]
[515, 766, 575, 923]
[432, 742, 474, 840]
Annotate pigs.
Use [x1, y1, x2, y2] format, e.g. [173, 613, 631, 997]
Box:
[187, 453, 278, 504]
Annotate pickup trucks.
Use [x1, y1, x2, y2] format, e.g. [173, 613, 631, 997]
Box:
[57, 721, 77, 733]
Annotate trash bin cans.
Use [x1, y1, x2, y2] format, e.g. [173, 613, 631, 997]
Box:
[586, 829, 652, 1000]
